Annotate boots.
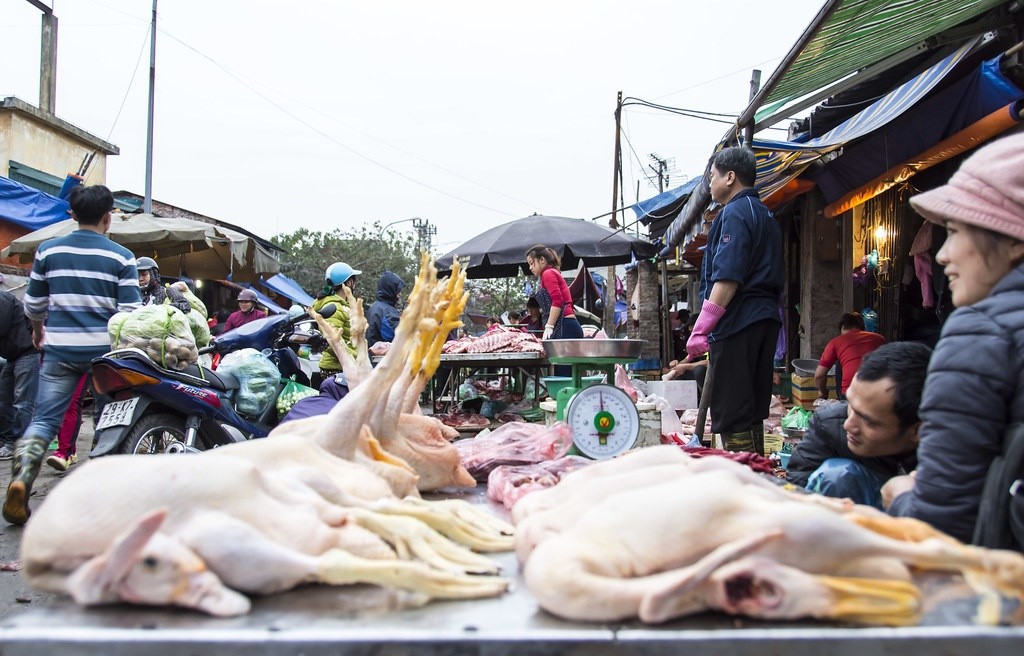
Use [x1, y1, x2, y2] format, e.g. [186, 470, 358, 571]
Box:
[720, 423, 765, 458]
[422, 384, 430, 405]
[2, 433, 49, 525]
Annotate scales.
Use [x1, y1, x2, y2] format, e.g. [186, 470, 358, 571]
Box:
[783, 427, 809, 441]
[504, 324, 529, 329]
[541, 338, 648, 460]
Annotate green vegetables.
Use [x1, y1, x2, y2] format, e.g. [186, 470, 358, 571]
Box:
[108, 292, 210, 349]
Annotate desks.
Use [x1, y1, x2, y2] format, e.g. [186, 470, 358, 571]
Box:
[371, 351, 545, 433]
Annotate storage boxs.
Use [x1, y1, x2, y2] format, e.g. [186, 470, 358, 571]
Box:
[646, 379, 698, 411]
[541, 375, 604, 401]
[539, 401, 662, 449]
[790, 371, 838, 411]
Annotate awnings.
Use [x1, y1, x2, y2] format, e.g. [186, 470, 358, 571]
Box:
[633, 0, 1024, 266]
[0, 175, 315, 313]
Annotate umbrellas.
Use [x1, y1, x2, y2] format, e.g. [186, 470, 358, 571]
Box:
[430, 212, 656, 281]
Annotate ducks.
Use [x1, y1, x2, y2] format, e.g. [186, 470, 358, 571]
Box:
[16, 248, 515, 618]
[510, 443, 1024, 629]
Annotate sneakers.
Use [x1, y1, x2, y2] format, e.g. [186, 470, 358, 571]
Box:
[67, 453, 78, 466]
[46, 450, 68, 471]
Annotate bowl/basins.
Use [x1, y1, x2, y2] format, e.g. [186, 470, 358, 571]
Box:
[792, 358, 836, 378]
[541, 376, 604, 400]
[777, 451, 792, 469]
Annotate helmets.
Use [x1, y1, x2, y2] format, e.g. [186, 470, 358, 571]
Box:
[136, 256, 159, 270]
[324, 262, 362, 287]
[236, 289, 258, 303]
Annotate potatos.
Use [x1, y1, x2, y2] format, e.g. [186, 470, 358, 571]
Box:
[111, 335, 198, 368]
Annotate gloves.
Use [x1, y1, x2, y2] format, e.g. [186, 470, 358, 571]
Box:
[542, 324, 555, 340]
[686, 298, 726, 360]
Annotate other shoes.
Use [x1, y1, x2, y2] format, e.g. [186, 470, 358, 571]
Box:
[779, 394, 789, 402]
[0, 440, 18, 460]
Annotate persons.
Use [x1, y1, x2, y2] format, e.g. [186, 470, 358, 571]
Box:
[0, 257, 544, 472]
[1, 184, 145, 525]
[527, 245, 584, 379]
[686, 148, 789, 456]
[779, 133, 1024, 555]
[669, 309, 710, 404]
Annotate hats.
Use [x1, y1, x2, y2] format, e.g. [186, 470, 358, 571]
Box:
[909, 129, 1024, 241]
[527, 297, 540, 308]
